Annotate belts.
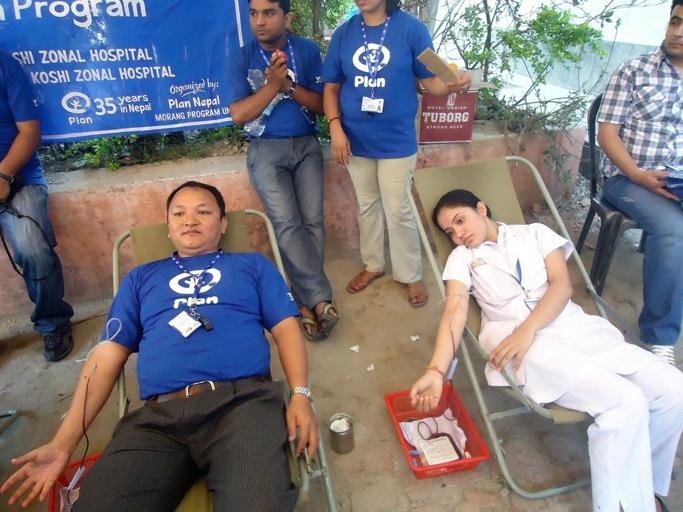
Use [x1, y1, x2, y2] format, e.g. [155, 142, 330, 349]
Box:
[155, 374, 271, 405]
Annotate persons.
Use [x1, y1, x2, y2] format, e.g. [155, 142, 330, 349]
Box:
[224, 0, 341, 343]
[0, 179, 321, 512]
[0, 50, 75, 362]
[594, 0, 682, 368]
[322, 0, 473, 310]
[408, 189, 682, 512]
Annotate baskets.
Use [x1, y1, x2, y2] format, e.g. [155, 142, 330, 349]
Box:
[384, 382, 491, 480]
[48, 452, 104, 512]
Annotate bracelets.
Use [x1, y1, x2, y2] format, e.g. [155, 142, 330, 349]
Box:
[327, 117, 341, 126]
[425, 366, 446, 377]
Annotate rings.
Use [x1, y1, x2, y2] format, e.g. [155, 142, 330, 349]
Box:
[464, 84, 468, 91]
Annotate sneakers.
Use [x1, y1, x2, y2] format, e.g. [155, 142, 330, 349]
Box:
[645, 343, 676, 367]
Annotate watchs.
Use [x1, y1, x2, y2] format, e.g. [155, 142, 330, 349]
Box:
[0, 173, 17, 185]
[287, 81, 297, 99]
[287, 386, 314, 402]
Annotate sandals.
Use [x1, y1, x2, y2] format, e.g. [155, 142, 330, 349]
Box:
[320, 299, 342, 333]
[407, 279, 429, 308]
[295, 315, 328, 342]
[346, 272, 386, 294]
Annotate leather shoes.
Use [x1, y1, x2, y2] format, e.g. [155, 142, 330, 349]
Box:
[43, 318, 74, 361]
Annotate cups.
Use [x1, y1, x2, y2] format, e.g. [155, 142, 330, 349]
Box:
[326, 412, 355, 455]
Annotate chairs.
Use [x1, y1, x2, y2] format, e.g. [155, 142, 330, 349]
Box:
[572, 95, 649, 295]
[404, 156, 614, 498]
[111, 208, 337, 512]
